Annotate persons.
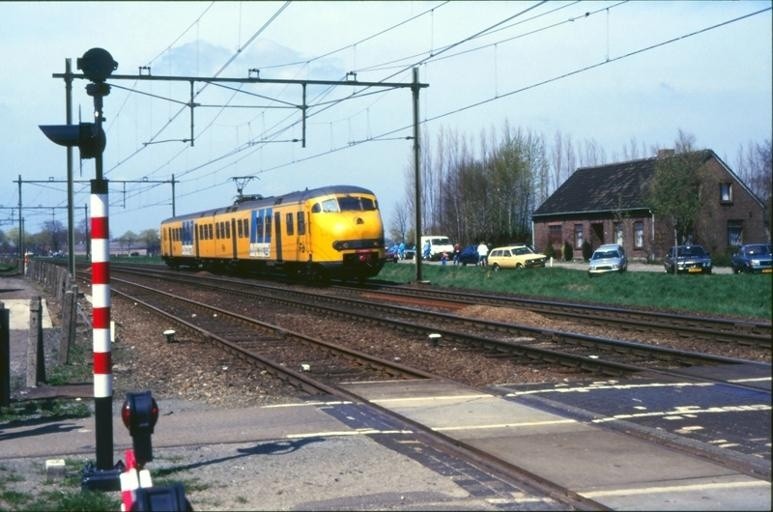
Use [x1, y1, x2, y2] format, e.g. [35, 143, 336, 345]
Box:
[451, 243, 461, 265]
[439, 249, 449, 265]
[476, 238, 489, 268]
[559, 240, 566, 262]
[423, 239, 432, 261]
[383, 239, 406, 262]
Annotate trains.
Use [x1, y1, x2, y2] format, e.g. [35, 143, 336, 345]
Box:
[158, 184, 386, 287]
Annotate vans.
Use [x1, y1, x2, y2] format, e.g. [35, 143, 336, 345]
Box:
[421, 234, 455, 262]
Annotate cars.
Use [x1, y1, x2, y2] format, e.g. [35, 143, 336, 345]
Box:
[732, 241, 772, 275]
[488, 244, 547, 273]
[384, 249, 398, 263]
[664, 245, 713, 276]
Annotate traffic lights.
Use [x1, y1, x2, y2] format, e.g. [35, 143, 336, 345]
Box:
[35, 120, 105, 160]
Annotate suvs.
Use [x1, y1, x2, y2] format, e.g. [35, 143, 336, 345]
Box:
[589, 243, 628, 278]
